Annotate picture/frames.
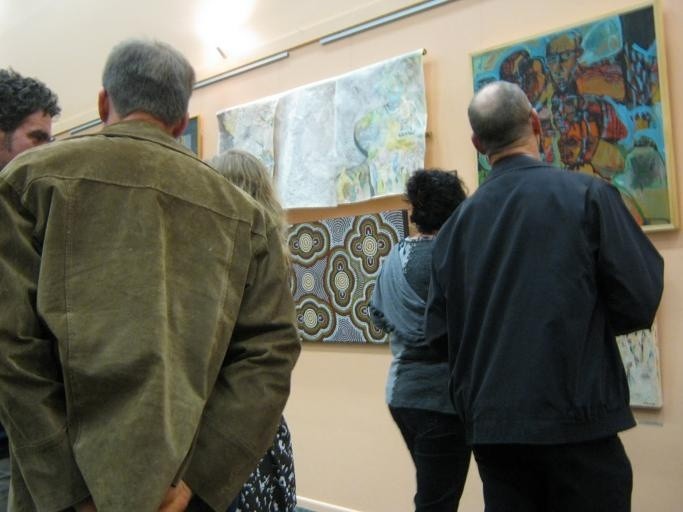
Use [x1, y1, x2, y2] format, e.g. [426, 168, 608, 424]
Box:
[466, 0, 682, 234]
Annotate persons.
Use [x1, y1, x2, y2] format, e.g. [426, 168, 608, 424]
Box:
[1, 69, 62, 511]
[425, 79, 665, 512]
[1, 38, 301, 512]
[187, 148, 298, 512]
[366, 167, 471, 512]
